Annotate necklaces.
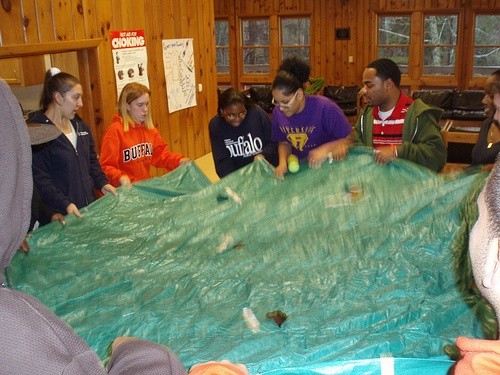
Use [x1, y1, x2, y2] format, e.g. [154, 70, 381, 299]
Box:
[487, 126, 493, 148]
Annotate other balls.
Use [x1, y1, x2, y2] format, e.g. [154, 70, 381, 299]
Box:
[288, 155, 299, 174]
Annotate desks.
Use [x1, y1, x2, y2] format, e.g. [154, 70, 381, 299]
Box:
[436, 120, 483, 147]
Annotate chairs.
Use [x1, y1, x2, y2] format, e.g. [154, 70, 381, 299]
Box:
[453, 89, 488, 118]
[414, 90, 452, 117]
[324, 85, 359, 125]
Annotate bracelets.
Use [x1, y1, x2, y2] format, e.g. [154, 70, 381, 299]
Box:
[394, 147, 398, 157]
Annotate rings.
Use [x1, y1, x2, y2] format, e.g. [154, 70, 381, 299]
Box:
[311, 160, 314, 162]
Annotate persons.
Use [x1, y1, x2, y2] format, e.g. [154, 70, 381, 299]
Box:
[304, 77, 325, 94]
[20, 218, 40, 253]
[0, 78, 183, 375]
[356, 89, 368, 118]
[250, 85, 275, 113]
[94, 82, 190, 197]
[187, 158, 500, 375]
[333, 58, 447, 172]
[208, 88, 278, 178]
[271, 58, 353, 177]
[473, 71, 500, 167]
[23, 68, 118, 227]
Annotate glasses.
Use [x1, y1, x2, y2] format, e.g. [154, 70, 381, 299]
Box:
[271, 91, 297, 108]
[223, 108, 248, 120]
